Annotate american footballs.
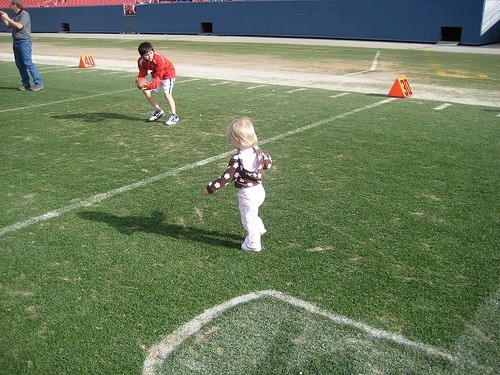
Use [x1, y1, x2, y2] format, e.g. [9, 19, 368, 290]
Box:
[136, 78, 147, 89]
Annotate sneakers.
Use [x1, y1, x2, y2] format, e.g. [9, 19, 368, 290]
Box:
[18, 85, 32, 91]
[29, 85, 44, 91]
[165, 112, 179, 126]
[148, 108, 164, 122]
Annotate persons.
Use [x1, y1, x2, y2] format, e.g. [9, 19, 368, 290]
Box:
[0, 0, 46, 93]
[135, 41, 182, 126]
[125, 1, 141, 15]
[203, 115, 272, 253]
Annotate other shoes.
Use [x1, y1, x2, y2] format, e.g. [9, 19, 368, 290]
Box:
[241, 243, 261, 252]
[261, 229, 267, 235]
